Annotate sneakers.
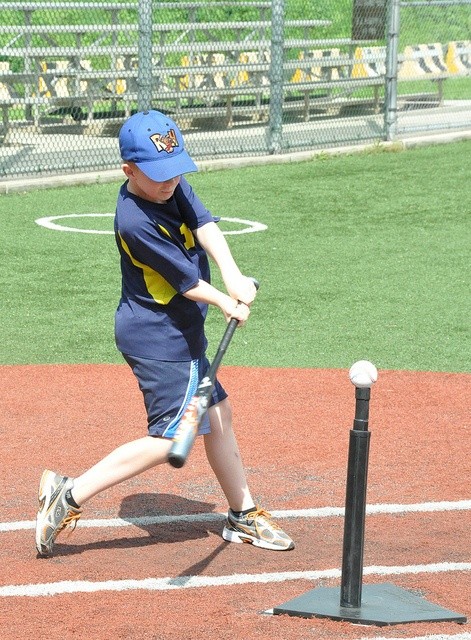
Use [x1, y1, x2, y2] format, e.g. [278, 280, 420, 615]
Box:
[34, 470, 84, 557]
[221, 509, 295, 551]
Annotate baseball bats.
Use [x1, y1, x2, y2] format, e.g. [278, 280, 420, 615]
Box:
[164, 274, 260, 469]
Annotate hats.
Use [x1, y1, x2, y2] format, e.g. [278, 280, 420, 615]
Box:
[119, 110, 199, 184]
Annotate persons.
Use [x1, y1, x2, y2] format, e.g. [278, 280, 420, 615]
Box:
[35, 105, 299, 559]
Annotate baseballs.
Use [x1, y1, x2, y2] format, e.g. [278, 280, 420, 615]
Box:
[348, 359, 379, 389]
